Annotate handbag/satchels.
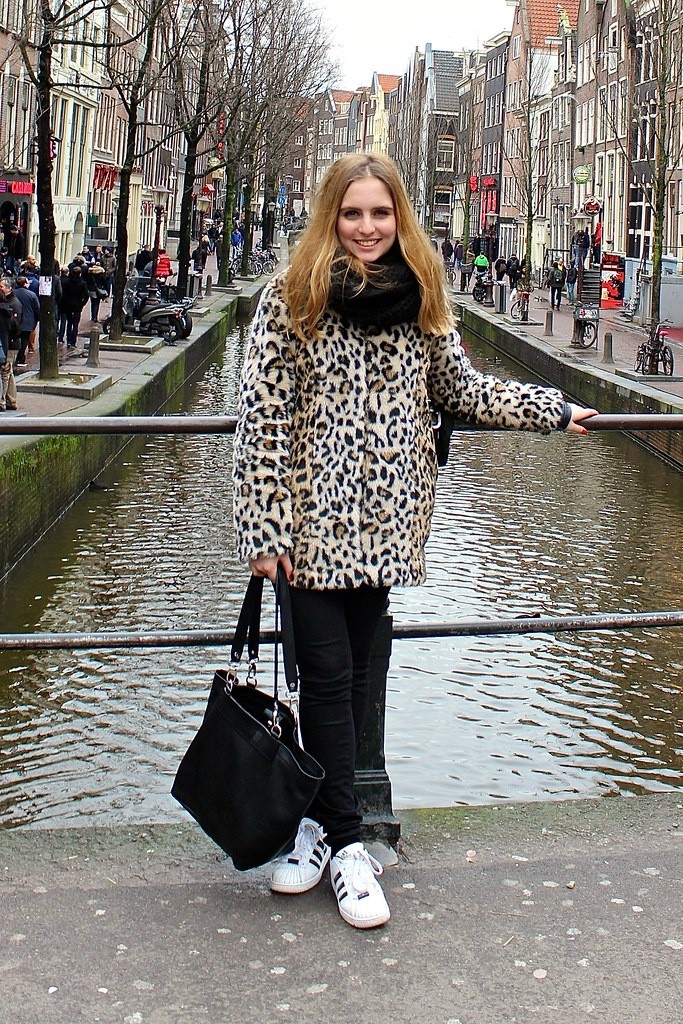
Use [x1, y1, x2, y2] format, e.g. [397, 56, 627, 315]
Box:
[96, 287, 108, 299]
[171, 560, 324, 872]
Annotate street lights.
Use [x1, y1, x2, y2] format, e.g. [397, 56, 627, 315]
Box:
[267, 201, 276, 247]
[570, 211, 591, 307]
[143, 185, 172, 305]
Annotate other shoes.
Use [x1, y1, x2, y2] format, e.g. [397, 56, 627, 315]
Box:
[567, 302, 573, 306]
[6, 404, 18, 411]
[557, 308, 559, 311]
[551, 306, 554, 309]
[70, 346, 77, 349]
[0, 406, 7, 412]
[16, 360, 27, 367]
[91, 317, 98, 323]
[28, 348, 35, 353]
[58, 341, 64, 344]
[67, 344, 71, 348]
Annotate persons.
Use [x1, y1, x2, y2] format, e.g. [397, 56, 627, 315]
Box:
[543, 257, 578, 311]
[466, 248, 476, 286]
[54, 244, 115, 350]
[569, 222, 601, 271]
[493, 253, 532, 291]
[44, 277, 50, 286]
[192, 222, 245, 275]
[300, 206, 308, 218]
[0, 225, 40, 412]
[231, 153, 596, 927]
[473, 251, 488, 272]
[284, 207, 295, 217]
[441, 240, 463, 270]
[214, 206, 244, 226]
[134, 244, 172, 281]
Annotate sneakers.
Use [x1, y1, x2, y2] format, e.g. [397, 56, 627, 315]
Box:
[327, 842, 390, 928]
[270, 818, 333, 892]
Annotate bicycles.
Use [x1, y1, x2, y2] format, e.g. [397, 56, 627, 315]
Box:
[460, 261, 475, 293]
[632, 317, 674, 376]
[445, 257, 457, 283]
[510, 280, 530, 320]
[226, 237, 279, 284]
[576, 300, 596, 348]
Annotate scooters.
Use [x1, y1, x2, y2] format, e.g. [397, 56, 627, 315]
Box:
[471, 269, 487, 302]
[103, 288, 197, 341]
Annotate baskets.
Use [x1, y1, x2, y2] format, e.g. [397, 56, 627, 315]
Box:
[575, 306, 599, 322]
[461, 264, 473, 274]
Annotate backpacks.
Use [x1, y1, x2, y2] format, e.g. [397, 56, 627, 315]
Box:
[553, 269, 563, 287]
[510, 259, 517, 272]
[498, 260, 507, 272]
